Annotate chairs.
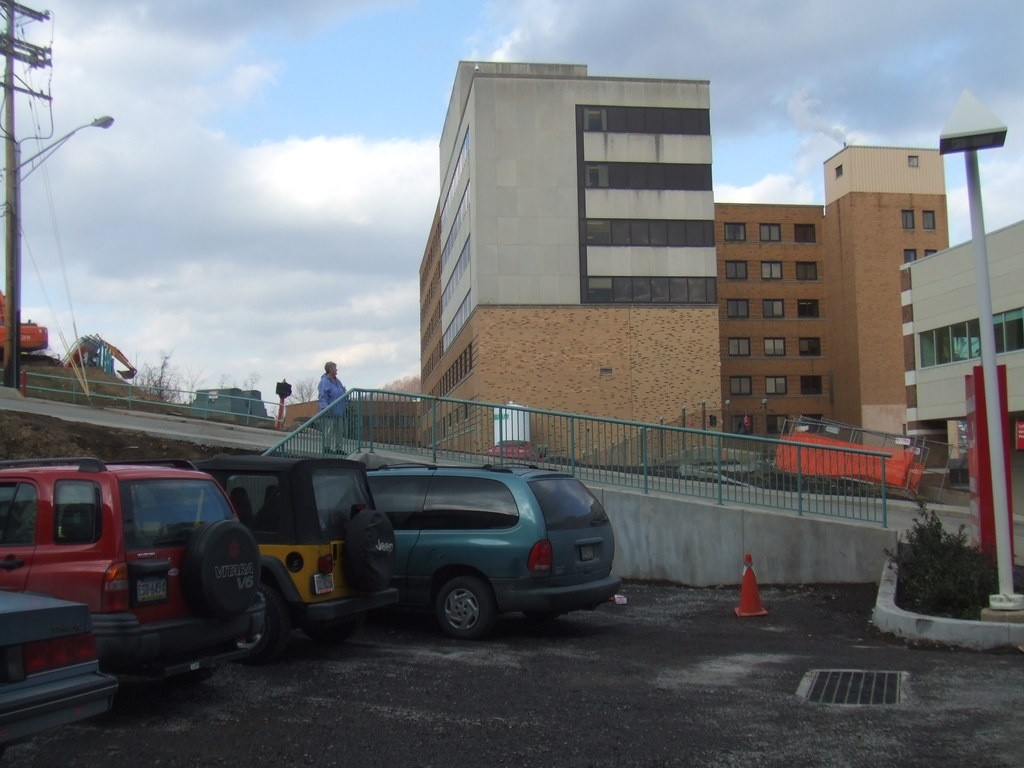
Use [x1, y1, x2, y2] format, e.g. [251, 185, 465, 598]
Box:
[231, 484, 279, 530]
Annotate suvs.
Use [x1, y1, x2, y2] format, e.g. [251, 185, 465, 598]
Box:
[344, 461, 623, 644]
[50, 448, 405, 649]
[0, 450, 271, 694]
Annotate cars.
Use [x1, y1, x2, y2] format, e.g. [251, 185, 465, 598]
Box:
[486, 439, 537, 461]
[0, 590, 120, 751]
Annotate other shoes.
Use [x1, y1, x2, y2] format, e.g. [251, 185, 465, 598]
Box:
[336, 450, 347, 455]
[323, 449, 335, 454]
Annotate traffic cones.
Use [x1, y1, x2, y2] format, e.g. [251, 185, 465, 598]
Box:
[733, 549, 770, 618]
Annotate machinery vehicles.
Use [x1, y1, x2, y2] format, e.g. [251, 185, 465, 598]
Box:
[0, 290, 137, 380]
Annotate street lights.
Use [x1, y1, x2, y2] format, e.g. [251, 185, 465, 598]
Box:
[938, 89, 1023, 613]
[4, 116, 117, 389]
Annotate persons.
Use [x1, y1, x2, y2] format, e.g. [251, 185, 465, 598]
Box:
[318, 362, 349, 456]
